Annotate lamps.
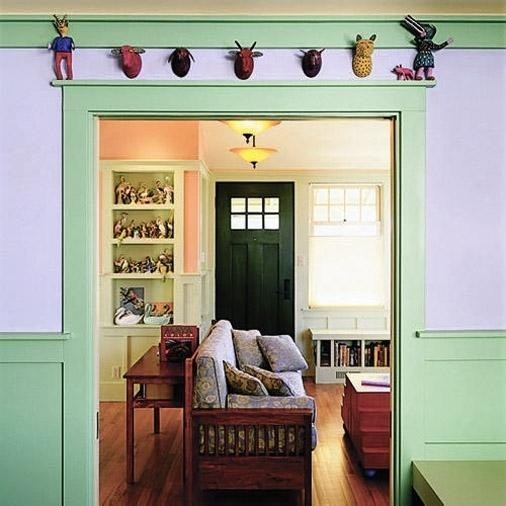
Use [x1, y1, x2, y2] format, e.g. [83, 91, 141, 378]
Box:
[221, 120, 282, 170]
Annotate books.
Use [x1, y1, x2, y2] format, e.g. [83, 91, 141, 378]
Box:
[335, 343, 389, 367]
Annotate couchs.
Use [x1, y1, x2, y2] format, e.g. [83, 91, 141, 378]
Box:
[186, 319, 317, 506]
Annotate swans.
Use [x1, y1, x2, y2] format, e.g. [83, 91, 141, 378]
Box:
[113, 307, 144, 325]
[143, 302, 170, 326]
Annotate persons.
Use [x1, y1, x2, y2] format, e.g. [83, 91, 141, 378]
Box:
[48, 36, 75, 80]
[413, 24, 453, 80]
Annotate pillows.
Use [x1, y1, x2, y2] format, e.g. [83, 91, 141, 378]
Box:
[221, 327, 309, 396]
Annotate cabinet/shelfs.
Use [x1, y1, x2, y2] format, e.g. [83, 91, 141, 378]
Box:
[100, 168, 184, 329]
[308, 328, 391, 385]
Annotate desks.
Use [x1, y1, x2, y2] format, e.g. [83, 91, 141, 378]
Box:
[122, 345, 200, 486]
